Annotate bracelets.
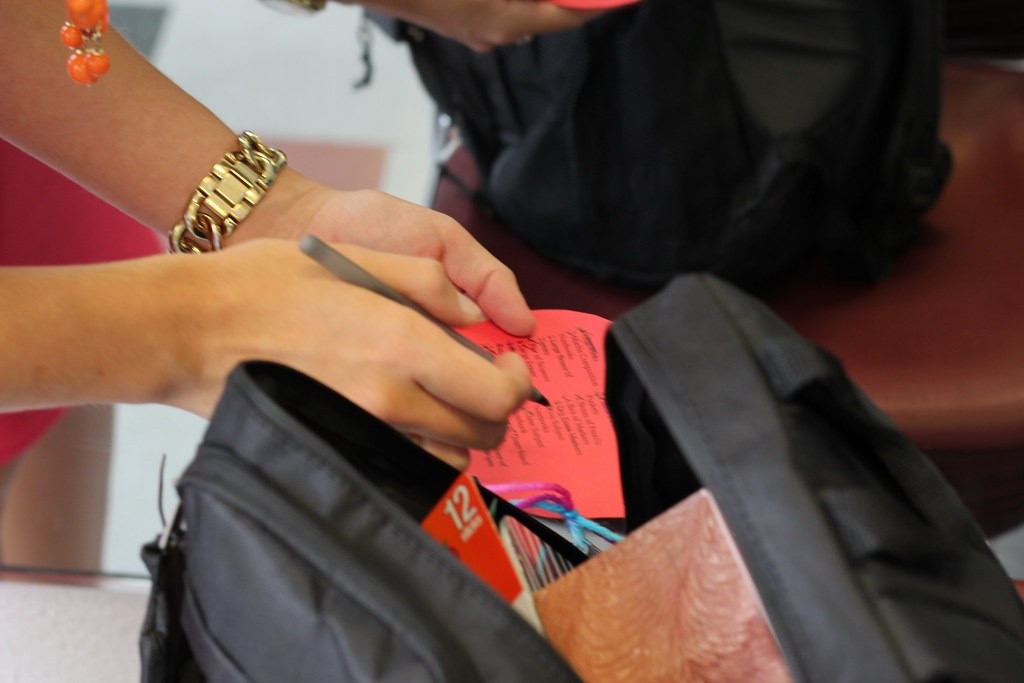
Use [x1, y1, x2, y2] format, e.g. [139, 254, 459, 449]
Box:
[163, 130, 289, 255]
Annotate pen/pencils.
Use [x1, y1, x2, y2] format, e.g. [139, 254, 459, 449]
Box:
[300, 236, 554, 410]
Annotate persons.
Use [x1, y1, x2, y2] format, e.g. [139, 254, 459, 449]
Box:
[0, 0, 619, 587]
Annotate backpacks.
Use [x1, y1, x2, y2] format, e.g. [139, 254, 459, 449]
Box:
[136, 276, 1024, 683]
[371, 0, 951, 305]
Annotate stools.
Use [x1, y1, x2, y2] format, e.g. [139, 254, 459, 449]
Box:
[433, 59, 1023, 452]
